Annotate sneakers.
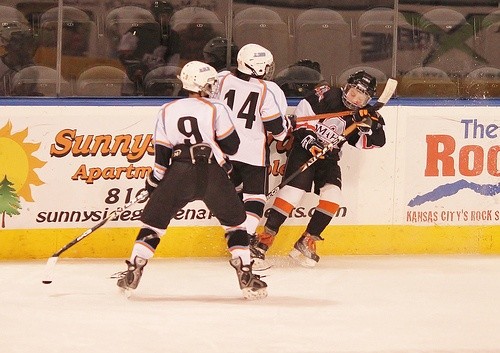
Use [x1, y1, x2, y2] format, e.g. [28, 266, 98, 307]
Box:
[228, 256, 268, 299]
[249, 233, 273, 270]
[111, 255, 148, 297]
[289, 231, 324, 269]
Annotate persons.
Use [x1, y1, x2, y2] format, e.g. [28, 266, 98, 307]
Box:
[3, 6, 240, 100]
[118, 0, 176, 94]
[116, 44, 386, 297]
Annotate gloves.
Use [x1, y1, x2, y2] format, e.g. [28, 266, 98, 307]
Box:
[298, 130, 327, 159]
[128, 64, 147, 83]
[145, 170, 160, 196]
[352, 107, 376, 135]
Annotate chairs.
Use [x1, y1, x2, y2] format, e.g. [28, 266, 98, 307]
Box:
[0, 5, 500, 99]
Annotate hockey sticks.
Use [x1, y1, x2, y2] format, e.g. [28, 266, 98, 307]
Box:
[297, 77, 399, 122]
[45, 190, 149, 270]
[266, 120, 364, 200]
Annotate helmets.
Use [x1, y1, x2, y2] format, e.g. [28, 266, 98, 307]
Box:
[181, 61, 218, 93]
[341, 71, 377, 109]
[203, 37, 235, 66]
[237, 44, 274, 79]
[0, 21, 35, 66]
[150, 0, 173, 26]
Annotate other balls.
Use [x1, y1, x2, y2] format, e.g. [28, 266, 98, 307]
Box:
[42, 281, 52, 284]
